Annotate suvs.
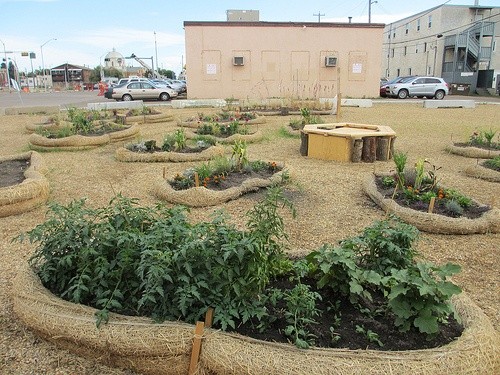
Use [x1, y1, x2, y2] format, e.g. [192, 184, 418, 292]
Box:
[380, 75, 422, 98]
[390, 76, 450, 100]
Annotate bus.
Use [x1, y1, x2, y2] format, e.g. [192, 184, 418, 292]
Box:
[50, 69, 83, 82]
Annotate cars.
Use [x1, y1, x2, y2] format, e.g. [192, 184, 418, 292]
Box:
[111, 81, 179, 102]
[104, 76, 187, 99]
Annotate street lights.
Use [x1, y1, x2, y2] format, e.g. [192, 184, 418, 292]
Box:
[369, 0, 378, 23]
[40, 38, 57, 76]
